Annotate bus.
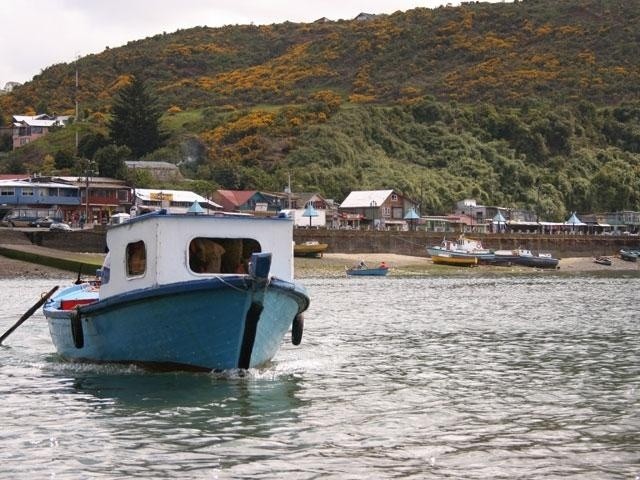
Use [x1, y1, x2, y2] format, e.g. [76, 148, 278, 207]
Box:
[1, 208, 63, 227]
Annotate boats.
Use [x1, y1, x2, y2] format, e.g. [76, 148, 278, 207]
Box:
[344, 261, 391, 276]
[618, 247, 640, 262]
[591, 255, 614, 265]
[294, 240, 330, 259]
[423, 231, 560, 270]
[38, 207, 314, 374]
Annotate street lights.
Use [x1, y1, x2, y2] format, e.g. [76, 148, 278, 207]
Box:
[204, 193, 213, 215]
[506, 205, 513, 234]
[78, 168, 95, 227]
[369, 200, 377, 231]
[469, 204, 474, 232]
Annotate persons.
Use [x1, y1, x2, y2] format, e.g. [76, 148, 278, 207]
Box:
[380, 262, 387, 267]
[357, 260, 368, 269]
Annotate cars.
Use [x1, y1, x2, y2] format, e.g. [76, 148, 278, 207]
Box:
[31, 217, 57, 228]
